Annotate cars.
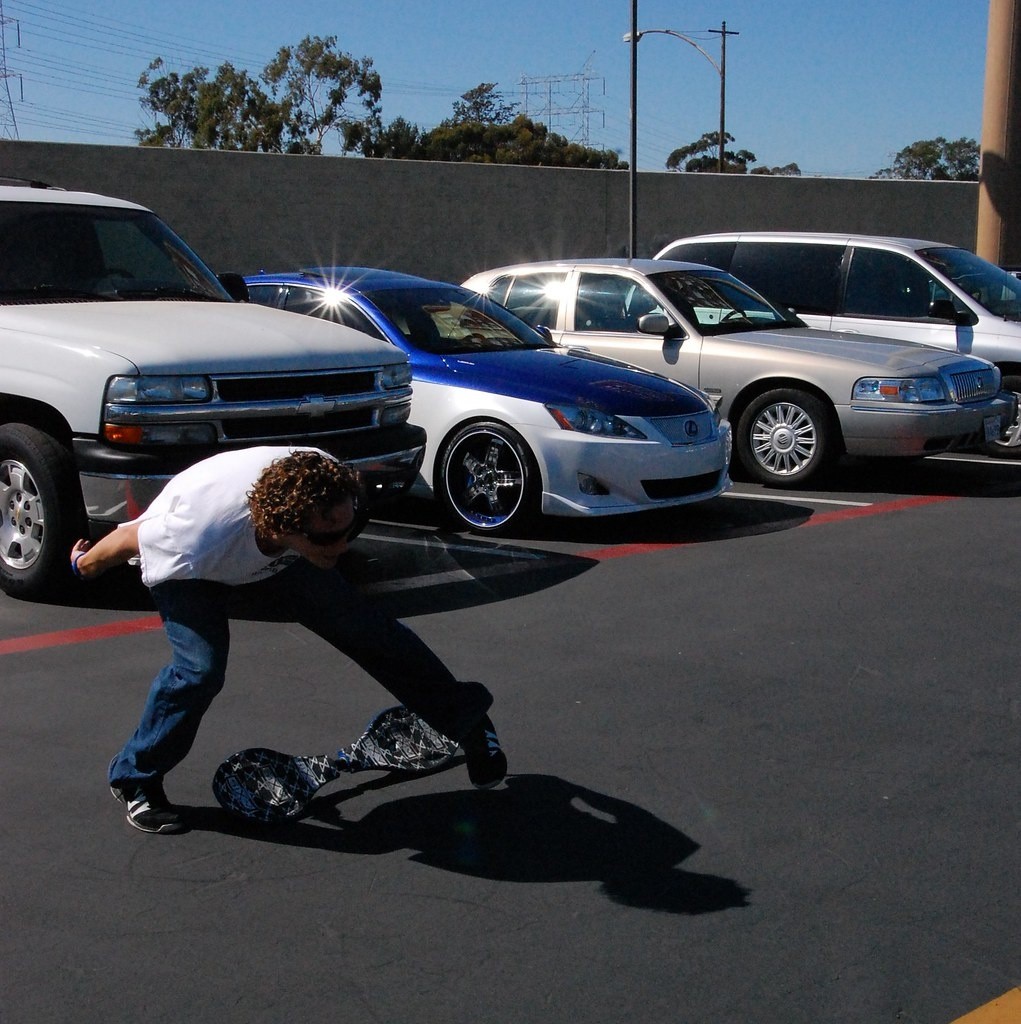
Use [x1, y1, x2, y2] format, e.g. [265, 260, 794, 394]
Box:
[453, 257, 1017, 489]
[216, 268, 732, 538]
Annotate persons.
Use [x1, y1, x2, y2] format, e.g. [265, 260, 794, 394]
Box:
[69, 446, 507, 833]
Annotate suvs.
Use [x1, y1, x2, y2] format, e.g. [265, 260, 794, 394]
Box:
[0, 180, 426, 592]
[618, 232, 1021, 459]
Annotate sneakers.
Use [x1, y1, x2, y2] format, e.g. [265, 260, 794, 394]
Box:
[458, 714, 507, 790]
[108, 752, 183, 830]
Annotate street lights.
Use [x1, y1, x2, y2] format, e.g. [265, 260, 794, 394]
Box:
[621, 28, 726, 173]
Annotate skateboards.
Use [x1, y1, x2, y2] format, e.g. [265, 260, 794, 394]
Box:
[211, 703, 467, 829]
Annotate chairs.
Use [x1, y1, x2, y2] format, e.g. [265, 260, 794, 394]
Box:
[402, 305, 442, 348]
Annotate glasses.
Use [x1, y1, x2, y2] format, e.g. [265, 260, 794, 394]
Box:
[298, 495, 370, 546]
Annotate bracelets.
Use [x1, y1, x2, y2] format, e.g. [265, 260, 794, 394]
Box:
[71, 552, 99, 581]
